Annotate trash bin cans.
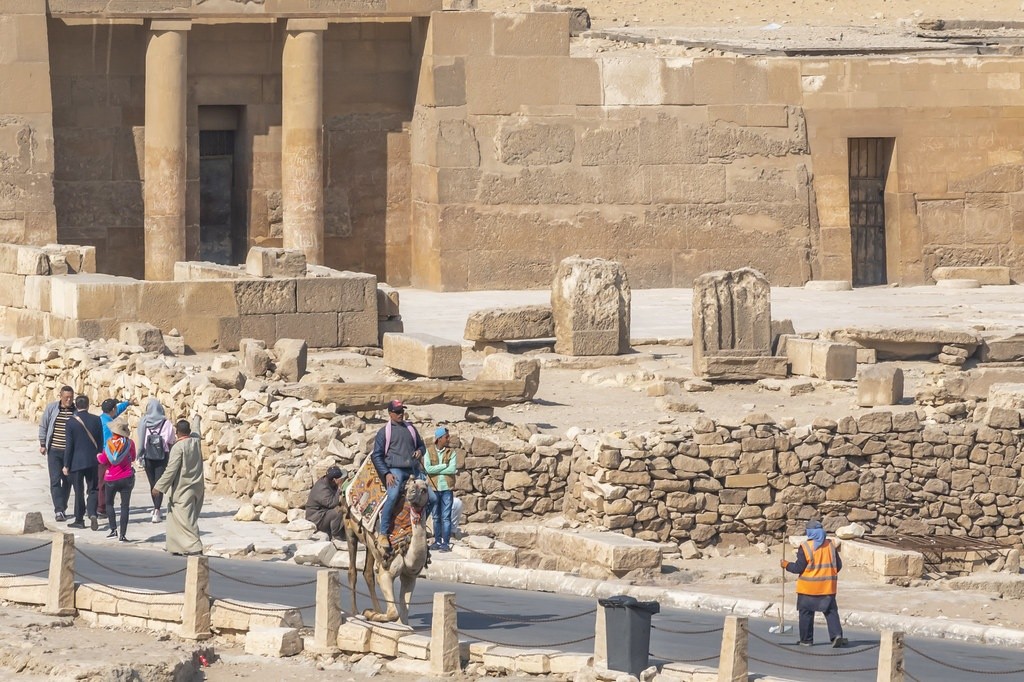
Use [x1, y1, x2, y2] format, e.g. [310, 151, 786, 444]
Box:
[598, 596, 660, 679]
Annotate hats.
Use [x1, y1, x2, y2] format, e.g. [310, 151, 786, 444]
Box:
[102, 399, 118, 412]
[107, 417, 131, 437]
[388, 400, 406, 411]
[326, 466, 348, 479]
[806, 519, 823, 529]
[435, 428, 448, 444]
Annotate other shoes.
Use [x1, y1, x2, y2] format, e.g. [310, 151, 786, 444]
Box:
[377, 534, 389, 548]
[832, 636, 843, 648]
[440, 541, 448, 550]
[119, 536, 129, 542]
[796, 639, 812, 646]
[152, 509, 162, 523]
[91, 516, 98, 530]
[56, 512, 66, 521]
[67, 520, 84, 528]
[333, 533, 347, 541]
[106, 531, 117, 538]
[429, 541, 442, 550]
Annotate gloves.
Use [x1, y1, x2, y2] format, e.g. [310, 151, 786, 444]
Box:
[781, 559, 789, 568]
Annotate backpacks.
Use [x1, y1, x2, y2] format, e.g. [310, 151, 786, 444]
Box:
[143, 420, 165, 460]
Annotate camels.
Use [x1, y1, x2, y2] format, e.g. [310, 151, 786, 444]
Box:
[339, 450, 430, 625]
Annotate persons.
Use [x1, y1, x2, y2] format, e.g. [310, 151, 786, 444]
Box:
[96, 397, 136, 519]
[38, 386, 77, 521]
[152, 420, 205, 556]
[137, 399, 175, 522]
[62, 396, 104, 530]
[305, 466, 345, 540]
[781, 520, 843, 648]
[424, 427, 457, 552]
[97, 417, 136, 543]
[371, 400, 426, 548]
